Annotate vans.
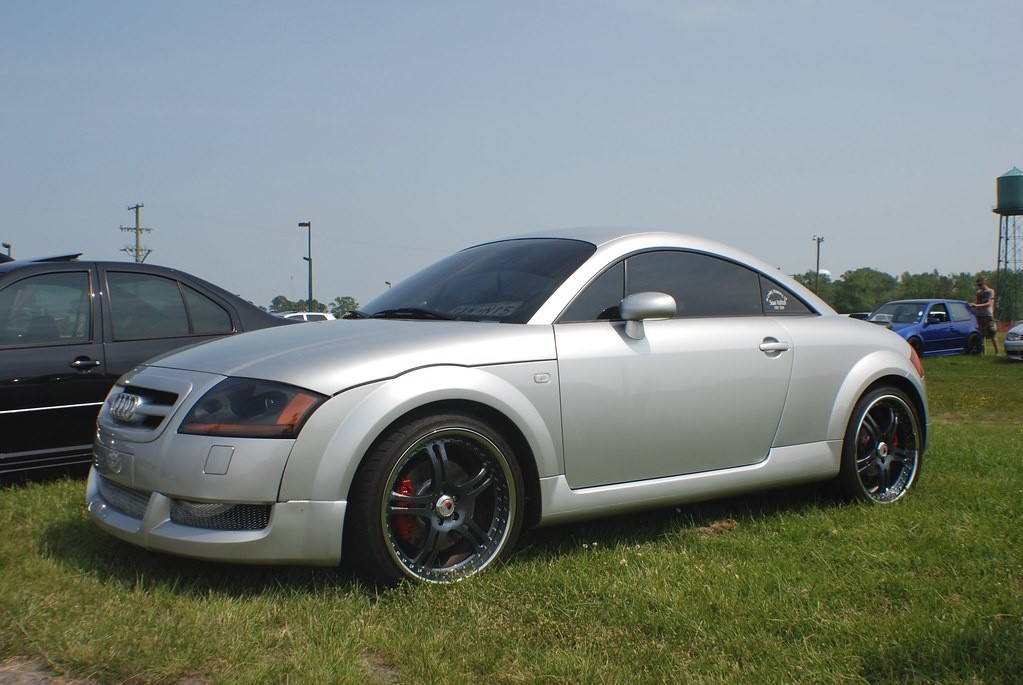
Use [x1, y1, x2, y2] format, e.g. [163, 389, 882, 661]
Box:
[273, 310, 337, 323]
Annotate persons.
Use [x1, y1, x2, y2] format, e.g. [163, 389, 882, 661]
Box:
[968, 277, 1001, 359]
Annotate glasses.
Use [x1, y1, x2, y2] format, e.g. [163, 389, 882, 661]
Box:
[977, 283, 984, 286]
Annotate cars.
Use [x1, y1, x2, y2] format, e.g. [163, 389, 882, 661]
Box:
[1004, 324, 1023, 360]
[848, 312, 872, 321]
[864, 299, 985, 359]
[0, 253, 313, 490]
[85, 228, 928, 585]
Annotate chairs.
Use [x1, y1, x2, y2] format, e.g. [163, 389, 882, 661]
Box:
[933, 314, 945, 322]
[907, 313, 919, 321]
[25, 316, 63, 344]
